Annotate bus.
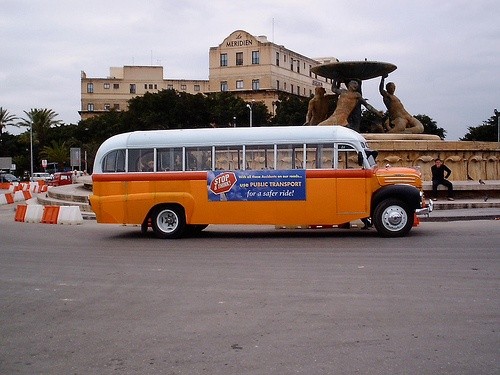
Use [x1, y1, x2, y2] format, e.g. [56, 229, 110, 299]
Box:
[90, 126, 436, 239]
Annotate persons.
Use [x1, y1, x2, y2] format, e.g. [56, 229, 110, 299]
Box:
[379, 73, 425, 134]
[344, 80, 363, 133]
[430, 158, 455, 202]
[317, 76, 389, 132]
[360, 217, 373, 230]
[303, 82, 342, 126]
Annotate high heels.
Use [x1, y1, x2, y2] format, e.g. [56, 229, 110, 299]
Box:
[361, 221, 372, 229]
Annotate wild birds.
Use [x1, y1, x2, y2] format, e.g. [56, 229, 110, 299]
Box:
[479, 179, 485, 184]
[484, 193, 489, 201]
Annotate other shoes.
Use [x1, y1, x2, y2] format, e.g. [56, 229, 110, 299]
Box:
[432, 197, 437, 201]
[448, 197, 455, 201]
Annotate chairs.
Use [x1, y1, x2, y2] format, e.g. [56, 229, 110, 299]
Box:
[117, 166, 302, 173]
